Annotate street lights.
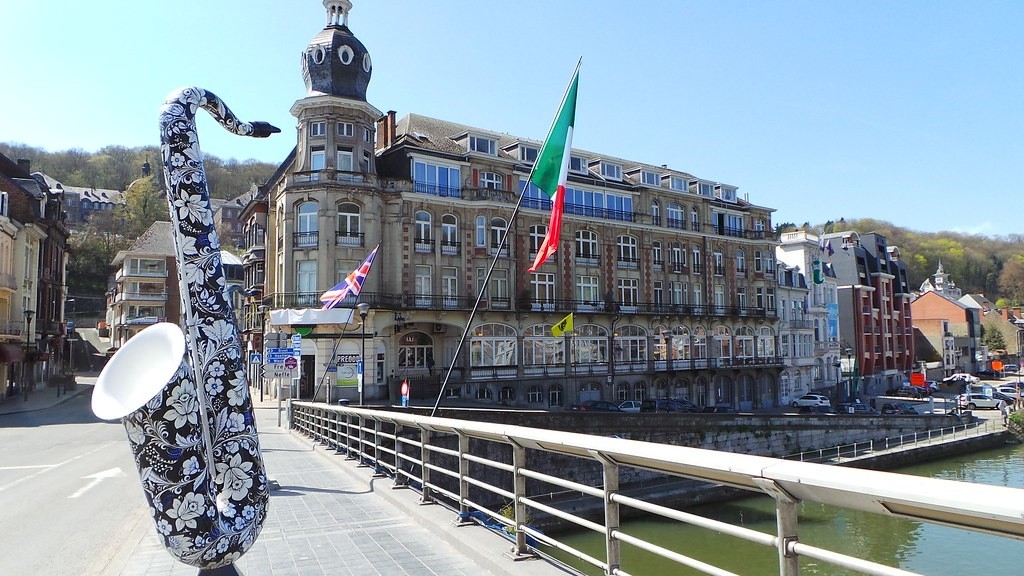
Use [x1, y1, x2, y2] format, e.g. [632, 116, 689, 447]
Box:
[954, 348, 963, 414]
[258, 303, 271, 402]
[356, 303, 372, 405]
[845, 346, 853, 404]
[23, 309, 36, 402]
[1016, 349, 1021, 398]
[123, 324, 129, 341]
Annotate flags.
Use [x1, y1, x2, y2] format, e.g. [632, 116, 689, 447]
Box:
[550, 313, 574, 337]
[527, 59, 579, 273]
[319, 244, 380, 310]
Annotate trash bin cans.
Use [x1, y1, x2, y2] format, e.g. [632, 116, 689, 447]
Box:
[338, 398, 350, 405]
[572, 402, 579, 411]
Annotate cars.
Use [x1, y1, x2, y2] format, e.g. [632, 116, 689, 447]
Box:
[1000, 364, 1020, 373]
[792, 394, 831, 408]
[672, 399, 704, 412]
[995, 387, 1024, 398]
[993, 391, 1014, 404]
[611, 400, 642, 414]
[885, 380, 940, 397]
[977, 369, 1000, 376]
[943, 373, 980, 384]
[955, 393, 1006, 409]
[700, 404, 738, 413]
[570, 400, 627, 413]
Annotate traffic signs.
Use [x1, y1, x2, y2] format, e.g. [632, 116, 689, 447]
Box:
[291, 334, 301, 356]
[264, 348, 294, 364]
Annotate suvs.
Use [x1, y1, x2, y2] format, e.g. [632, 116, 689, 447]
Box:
[640, 398, 686, 414]
[835, 403, 877, 414]
[881, 402, 918, 415]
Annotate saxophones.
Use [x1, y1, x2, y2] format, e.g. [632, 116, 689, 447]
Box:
[90, 82, 283, 572]
